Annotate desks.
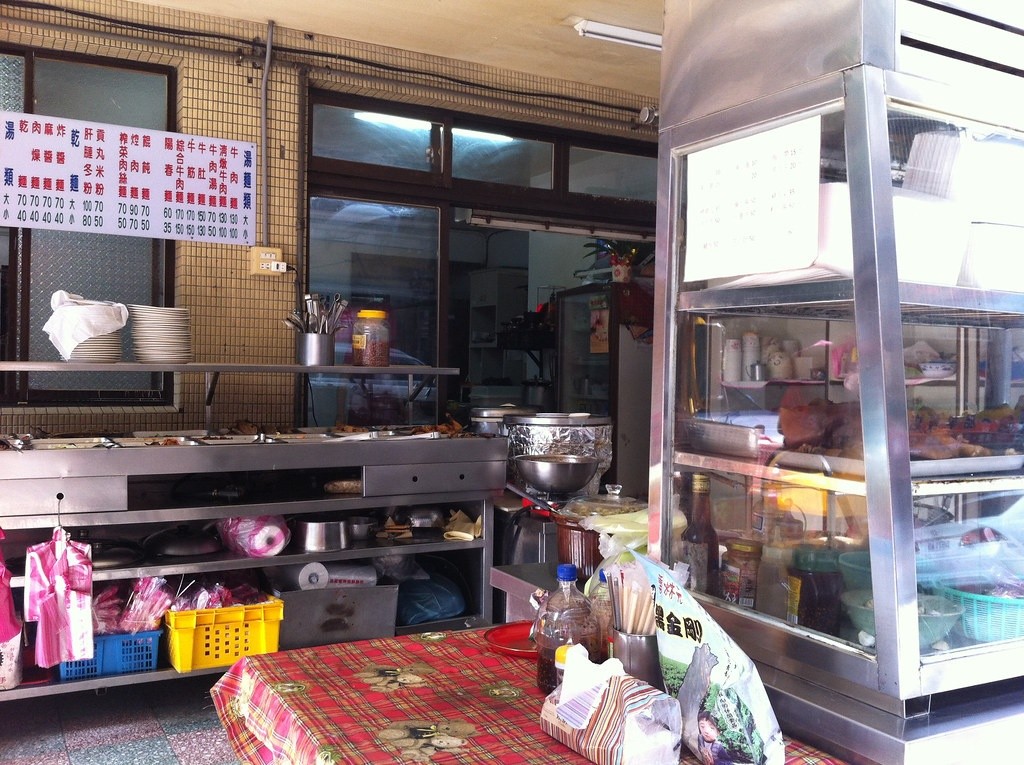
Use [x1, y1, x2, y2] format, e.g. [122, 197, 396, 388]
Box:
[694, 409, 781, 435]
[489, 562, 592, 624]
[207, 619, 849, 765]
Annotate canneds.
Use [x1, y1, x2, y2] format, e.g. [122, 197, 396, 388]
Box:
[721, 537, 764, 610]
[785, 546, 844, 635]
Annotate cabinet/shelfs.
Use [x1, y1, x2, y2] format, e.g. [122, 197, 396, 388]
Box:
[467, 266, 528, 386]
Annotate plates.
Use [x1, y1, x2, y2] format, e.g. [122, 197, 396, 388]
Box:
[126, 304, 195, 364]
[484, 620, 539, 658]
[58, 330, 123, 363]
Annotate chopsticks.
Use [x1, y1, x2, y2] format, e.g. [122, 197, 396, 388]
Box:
[606, 563, 657, 635]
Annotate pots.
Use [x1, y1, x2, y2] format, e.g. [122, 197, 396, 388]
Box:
[503, 414, 613, 492]
[554, 484, 648, 579]
[470, 404, 540, 438]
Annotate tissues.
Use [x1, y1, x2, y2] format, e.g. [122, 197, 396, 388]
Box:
[538, 642, 684, 765]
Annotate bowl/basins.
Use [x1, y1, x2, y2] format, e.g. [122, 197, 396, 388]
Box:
[296, 521, 351, 553]
[347, 516, 378, 541]
[919, 362, 956, 379]
[742, 332, 798, 382]
[722, 339, 741, 382]
[838, 551, 871, 591]
[514, 456, 600, 492]
[836, 590, 967, 649]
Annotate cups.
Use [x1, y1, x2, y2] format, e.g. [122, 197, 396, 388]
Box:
[296, 333, 335, 367]
[612, 626, 660, 690]
[746, 361, 767, 381]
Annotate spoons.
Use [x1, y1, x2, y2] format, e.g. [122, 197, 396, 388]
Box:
[285, 311, 318, 333]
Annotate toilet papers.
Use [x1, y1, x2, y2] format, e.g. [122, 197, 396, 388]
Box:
[225, 519, 285, 556]
[265, 562, 329, 592]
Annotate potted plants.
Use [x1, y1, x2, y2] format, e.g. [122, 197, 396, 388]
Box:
[581, 236, 645, 283]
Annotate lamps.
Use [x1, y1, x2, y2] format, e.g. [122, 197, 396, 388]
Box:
[630, 105, 659, 130]
[573, 19, 663, 52]
[465, 208, 656, 244]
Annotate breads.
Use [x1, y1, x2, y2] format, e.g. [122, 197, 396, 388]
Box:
[234, 418, 369, 434]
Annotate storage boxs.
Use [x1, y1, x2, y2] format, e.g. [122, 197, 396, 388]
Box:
[58, 626, 164, 682]
[0, 610, 24, 692]
[164, 593, 284, 675]
[270, 580, 399, 652]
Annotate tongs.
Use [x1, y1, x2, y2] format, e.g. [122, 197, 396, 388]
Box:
[305, 293, 348, 335]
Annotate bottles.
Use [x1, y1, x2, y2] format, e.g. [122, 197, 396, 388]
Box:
[788, 548, 841, 638]
[352, 310, 391, 367]
[537, 564, 612, 694]
[721, 486, 804, 621]
[583, 378, 589, 393]
[682, 475, 719, 597]
[534, 375, 543, 386]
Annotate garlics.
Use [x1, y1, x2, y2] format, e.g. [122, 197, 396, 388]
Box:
[932, 640, 950, 651]
[858, 630, 876, 647]
[865, 597, 939, 614]
